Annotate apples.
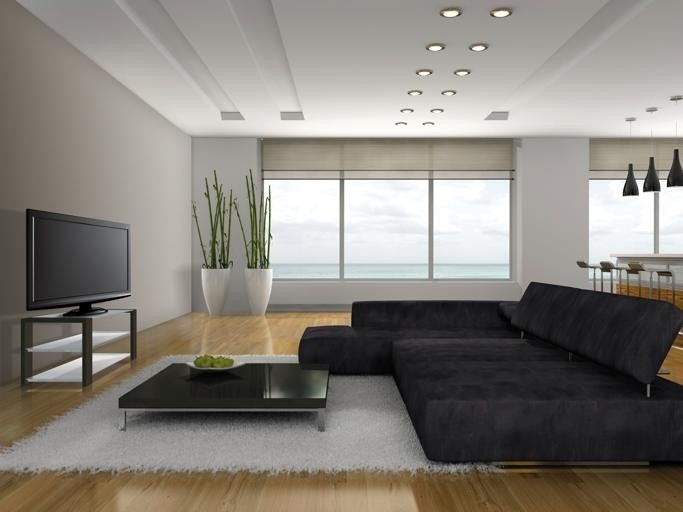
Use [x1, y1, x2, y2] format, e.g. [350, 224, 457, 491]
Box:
[194, 354, 234, 368]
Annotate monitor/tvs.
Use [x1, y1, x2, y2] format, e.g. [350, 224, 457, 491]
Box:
[25, 207, 132, 316]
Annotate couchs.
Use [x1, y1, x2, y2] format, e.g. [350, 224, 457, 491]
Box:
[296, 280, 683, 464]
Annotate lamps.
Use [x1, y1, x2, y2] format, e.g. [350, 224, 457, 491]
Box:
[665, 96, 683, 187]
[621, 117, 639, 197]
[642, 107, 661, 192]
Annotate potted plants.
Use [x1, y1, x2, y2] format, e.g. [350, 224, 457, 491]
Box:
[235, 168, 273, 315]
[191, 169, 235, 315]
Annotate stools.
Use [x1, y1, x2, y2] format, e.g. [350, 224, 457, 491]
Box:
[628, 262, 675, 304]
[577, 261, 614, 294]
[600, 261, 641, 298]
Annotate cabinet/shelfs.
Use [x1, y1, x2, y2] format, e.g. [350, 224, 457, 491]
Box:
[21, 309, 135, 386]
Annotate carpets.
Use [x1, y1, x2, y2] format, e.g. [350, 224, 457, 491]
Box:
[0, 354, 495, 473]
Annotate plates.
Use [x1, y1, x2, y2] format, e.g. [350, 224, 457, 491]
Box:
[185, 361, 247, 372]
